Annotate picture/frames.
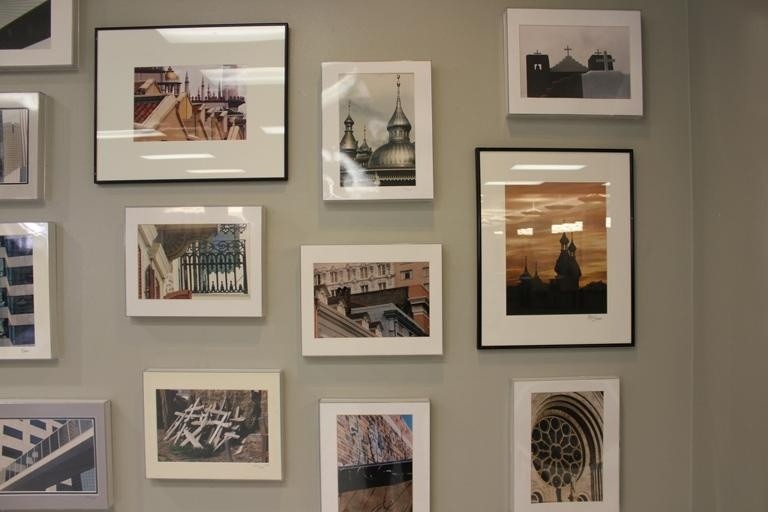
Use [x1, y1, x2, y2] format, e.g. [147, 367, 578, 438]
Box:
[142, 368, 285, 481]
[1, 222, 58, 361]
[0, 398, 114, 511]
[509, 376, 621, 512]
[321, 61, 435, 203]
[502, 7, 644, 121]
[0, 0, 81, 74]
[125, 205, 266, 319]
[0, 92, 46, 201]
[319, 397, 431, 511]
[300, 243, 444, 357]
[94, 22, 290, 185]
[475, 147, 635, 348]
